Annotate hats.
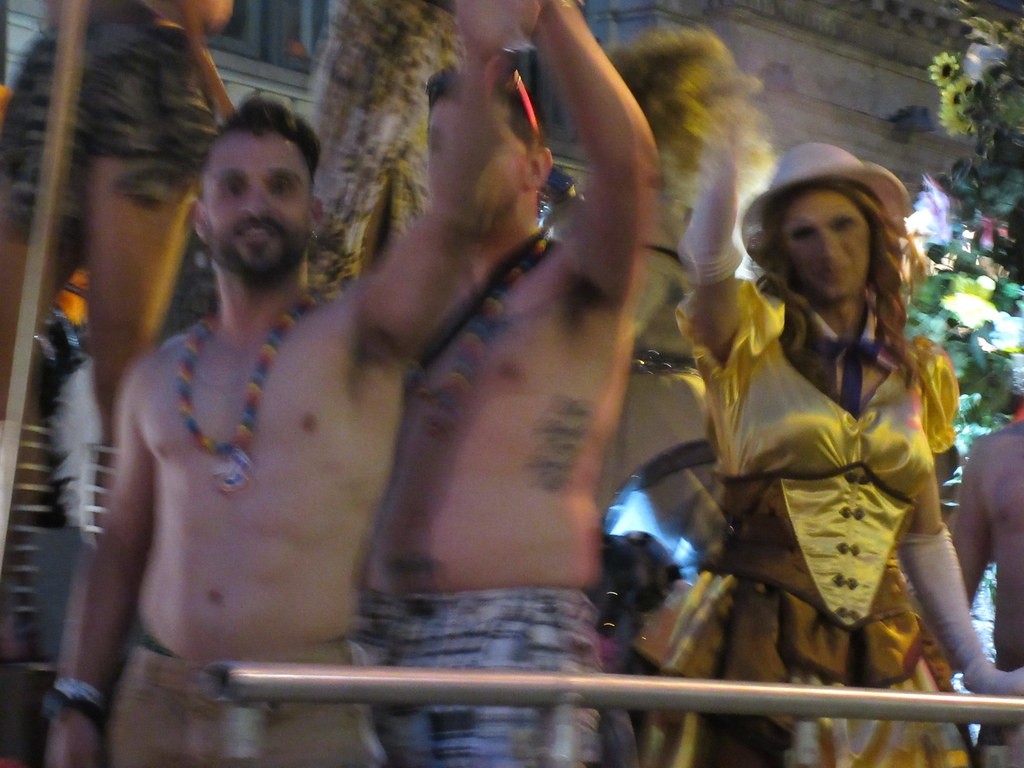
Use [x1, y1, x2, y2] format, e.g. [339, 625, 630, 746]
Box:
[739, 142, 913, 291]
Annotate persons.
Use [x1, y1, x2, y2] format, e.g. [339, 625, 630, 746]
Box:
[636, 142, 1024, 768]
[1, 0, 231, 766]
[922, 423, 1023, 766]
[44, 0, 542, 767]
[350, 2, 659, 768]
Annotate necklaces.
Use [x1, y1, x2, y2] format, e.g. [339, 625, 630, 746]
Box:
[407, 235, 555, 433]
[177, 301, 312, 492]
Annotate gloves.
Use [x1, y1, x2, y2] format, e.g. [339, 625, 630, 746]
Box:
[896, 521, 1024, 694]
[680, 150, 742, 283]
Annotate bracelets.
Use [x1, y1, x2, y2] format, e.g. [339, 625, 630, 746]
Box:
[51, 676, 108, 708]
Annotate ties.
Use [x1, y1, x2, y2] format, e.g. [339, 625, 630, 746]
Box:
[814, 337, 884, 421]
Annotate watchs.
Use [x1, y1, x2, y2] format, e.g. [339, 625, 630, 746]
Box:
[40, 691, 107, 730]
